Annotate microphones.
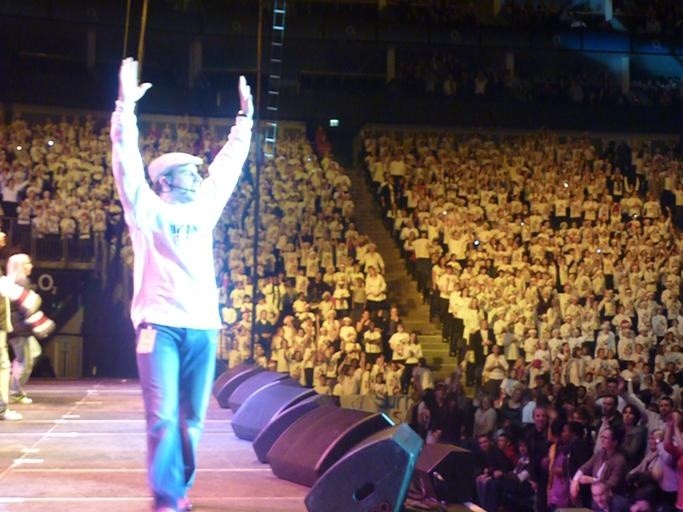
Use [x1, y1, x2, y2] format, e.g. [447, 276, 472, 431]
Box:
[167, 183, 196, 194]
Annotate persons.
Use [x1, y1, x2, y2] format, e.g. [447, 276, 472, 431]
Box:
[577, 371, 595, 393]
[653, 372, 674, 394]
[577, 384, 590, 409]
[526, 364, 563, 403]
[570, 426, 626, 512]
[475, 435, 509, 502]
[361, 352, 471, 447]
[620, 403, 647, 467]
[404, 242, 683, 364]
[362, 321, 384, 363]
[639, 362, 652, 406]
[497, 388, 524, 425]
[591, 393, 623, 458]
[471, 395, 497, 439]
[589, 479, 631, 512]
[627, 374, 673, 456]
[485, 433, 519, 509]
[621, 360, 639, 393]
[546, 420, 594, 488]
[510, 435, 538, 495]
[626, 471, 681, 512]
[605, 377, 626, 413]
[526, 404, 554, 512]
[388, 323, 411, 363]
[213, 185, 361, 411]
[108, 56, 256, 512]
[648, 379, 667, 413]
[373, 307, 384, 328]
[571, 406, 589, 424]
[5, 253, 57, 405]
[359, 119, 683, 243]
[663, 413, 683, 512]
[0, 107, 136, 268]
[385, 303, 403, 362]
[482, 364, 505, 398]
[556, 382, 578, 415]
[0, 230, 43, 422]
[138, 112, 353, 185]
[499, 367, 520, 399]
[356, 308, 374, 335]
[358, 242, 386, 276]
[364, 265, 389, 319]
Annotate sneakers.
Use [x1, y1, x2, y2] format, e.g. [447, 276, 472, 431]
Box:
[18, 396, 32, 405]
[0, 409, 23, 420]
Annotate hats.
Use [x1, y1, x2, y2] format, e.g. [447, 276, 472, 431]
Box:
[147, 152, 203, 185]
